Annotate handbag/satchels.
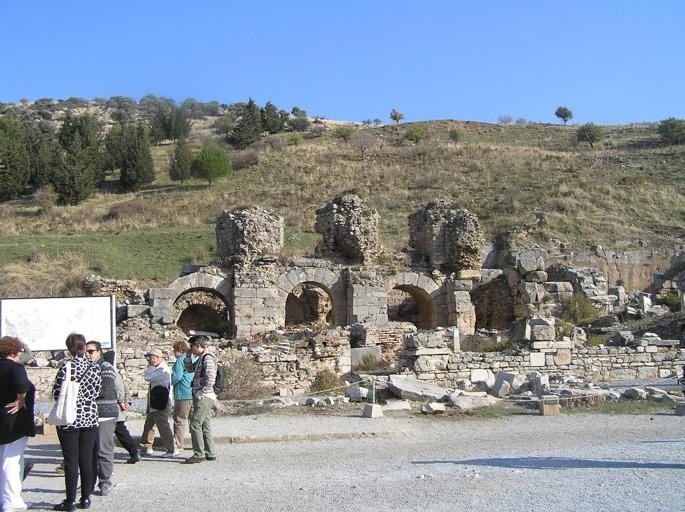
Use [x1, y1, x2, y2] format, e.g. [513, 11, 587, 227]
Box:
[46, 380, 79, 425]
[150, 386, 169, 410]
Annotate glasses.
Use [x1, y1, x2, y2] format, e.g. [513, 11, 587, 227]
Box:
[87, 350, 98, 354]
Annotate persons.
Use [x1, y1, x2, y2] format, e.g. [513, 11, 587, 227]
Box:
[50, 332, 102, 512]
[54, 459, 64, 474]
[170, 340, 197, 452]
[86, 340, 120, 495]
[0, 335, 35, 512]
[136, 348, 180, 457]
[103, 352, 140, 463]
[183, 336, 218, 463]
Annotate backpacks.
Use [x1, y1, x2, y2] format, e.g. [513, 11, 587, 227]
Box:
[202, 354, 224, 395]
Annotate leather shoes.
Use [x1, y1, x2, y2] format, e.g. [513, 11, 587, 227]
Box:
[79, 497, 91, 508]
[54, 499, 76, 512]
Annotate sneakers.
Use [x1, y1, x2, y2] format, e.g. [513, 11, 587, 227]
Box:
[100, 487, 112, 496]
[127, 446, 217, 464]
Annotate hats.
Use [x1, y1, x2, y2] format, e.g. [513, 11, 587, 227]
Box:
[144, 349, 162, 358]
